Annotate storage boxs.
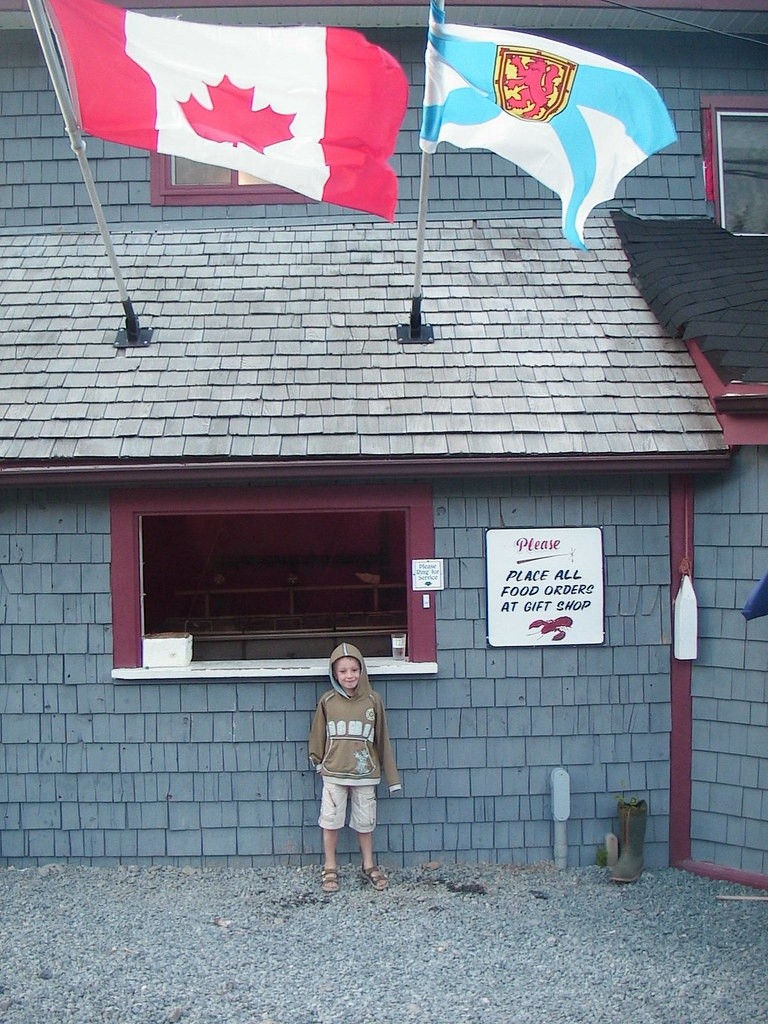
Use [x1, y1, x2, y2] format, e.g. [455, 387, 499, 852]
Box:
[167, 613, 408, 660]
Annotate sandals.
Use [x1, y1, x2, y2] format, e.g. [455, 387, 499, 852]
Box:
[322, 866, 339, 892]
[361, 862, 389, 890]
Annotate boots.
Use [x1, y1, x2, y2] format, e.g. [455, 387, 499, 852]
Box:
[609, 799, 647, 881]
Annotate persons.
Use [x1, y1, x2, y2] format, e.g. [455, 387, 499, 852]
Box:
[308, 643, 401, 891]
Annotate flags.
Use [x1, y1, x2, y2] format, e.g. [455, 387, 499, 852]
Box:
[418, 1, 678, 253]
[44, 0, 409, 226]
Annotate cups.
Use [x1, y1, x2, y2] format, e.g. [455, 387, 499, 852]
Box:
[391, 633, 406, 661]
